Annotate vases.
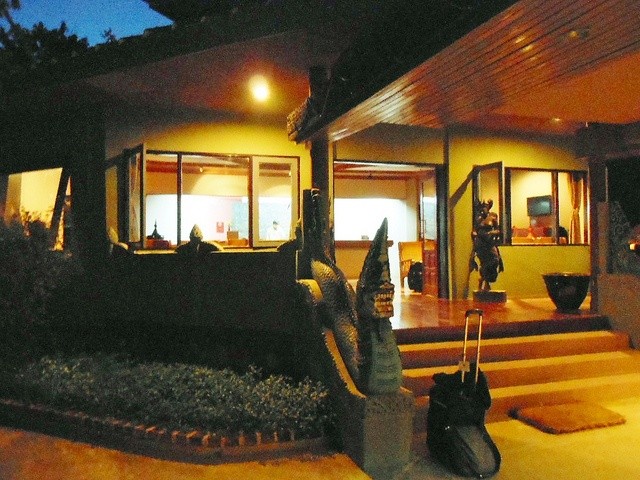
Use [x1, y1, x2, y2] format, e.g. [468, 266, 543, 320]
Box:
[540, 272, 591, 314]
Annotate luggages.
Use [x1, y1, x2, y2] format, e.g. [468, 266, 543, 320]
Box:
[426, 308, 501, 479]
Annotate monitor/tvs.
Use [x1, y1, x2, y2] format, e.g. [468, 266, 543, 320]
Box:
[527, 195, 552, 216]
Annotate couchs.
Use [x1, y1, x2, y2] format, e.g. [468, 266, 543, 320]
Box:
[397, 241, 435, 288]
